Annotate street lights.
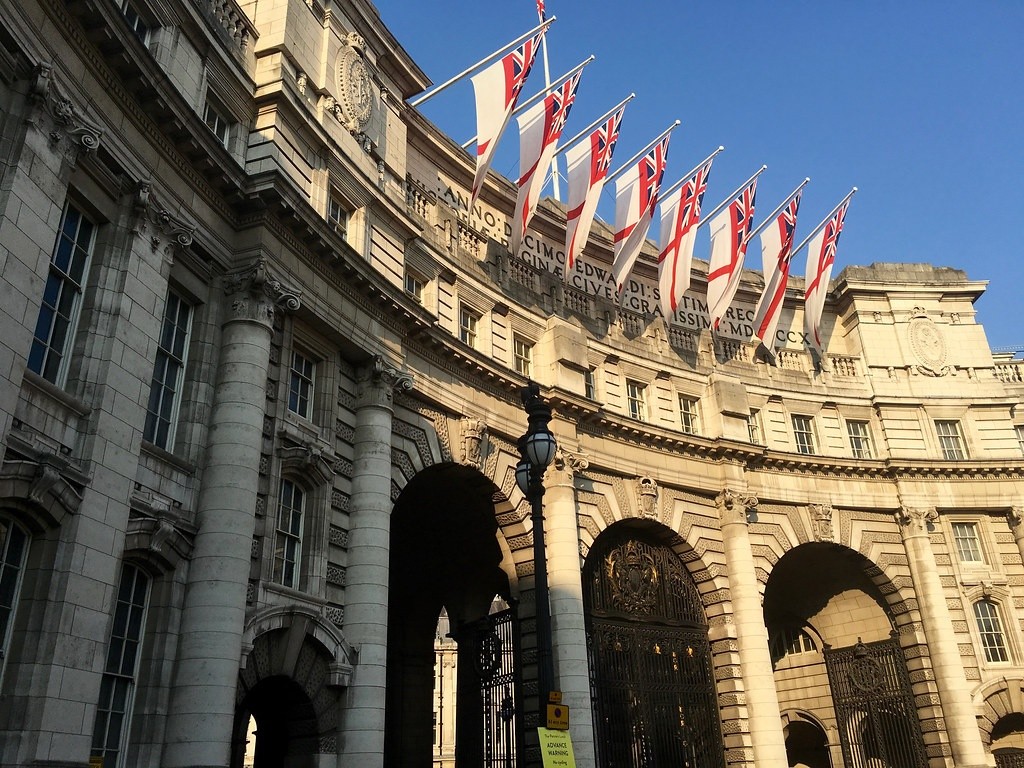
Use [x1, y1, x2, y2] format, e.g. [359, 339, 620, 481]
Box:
[515, 373, 558, 724]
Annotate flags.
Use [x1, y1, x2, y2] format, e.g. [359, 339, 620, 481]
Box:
[536, 0, 548, 26]
[612, 132, 671, 314]
[658, 157, 715, 328]
[512, 67, 584, 260]
[562, 103, 627, 287]
[705, 177, 758, 343]
[465, 21, 551, 225]
[753, 187, 804, 356]
[802, 195, 851, 360]
